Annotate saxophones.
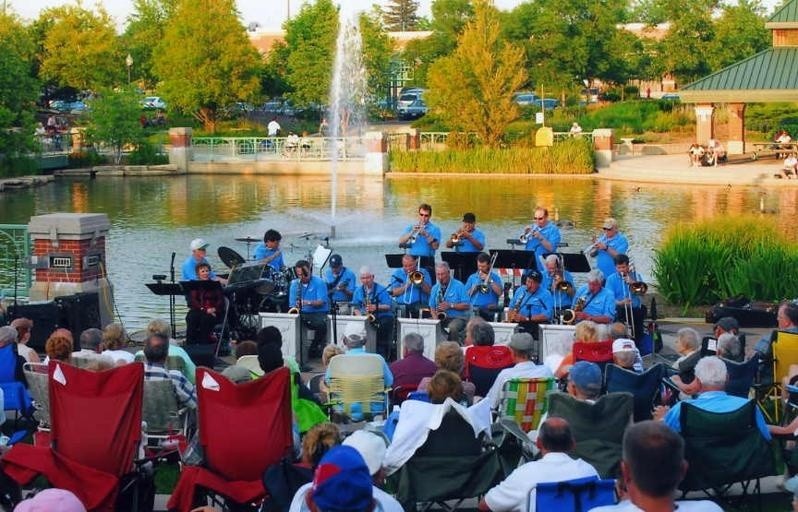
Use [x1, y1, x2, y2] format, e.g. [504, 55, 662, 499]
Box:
[501, 286, 529, 322]
[558, 290, 594, 325]
[288, 277, 305, 320]
[436, 279, 450, 333]
[366, 284, 381, 329]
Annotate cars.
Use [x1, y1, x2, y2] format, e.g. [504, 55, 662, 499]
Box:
[513, 94, 558, 110]
[662, 94, 679, 99]
[139, 96, 167, 111]
[598, 94, 621, 102]
[397, 87, 430, 120]
[580, 88, 598, 103]
[226, 98, 318, 115]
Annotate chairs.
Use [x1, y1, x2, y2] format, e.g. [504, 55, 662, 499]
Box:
[1, 297, 798, 511]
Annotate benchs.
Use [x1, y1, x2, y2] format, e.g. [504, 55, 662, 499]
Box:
[688, 133, 797, 165]
[284, 139, 325, 157]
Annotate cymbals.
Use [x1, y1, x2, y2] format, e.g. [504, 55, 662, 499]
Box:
[234, 236, 260, 242]
[297, 233, 313, 238]
[218, 247, 245, 269]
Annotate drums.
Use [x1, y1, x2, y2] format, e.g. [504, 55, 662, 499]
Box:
[254, 263, 276, 294]
[272, 272, 288, 298]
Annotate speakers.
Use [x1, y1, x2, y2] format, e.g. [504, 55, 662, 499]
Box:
[178, 343, 214, 368]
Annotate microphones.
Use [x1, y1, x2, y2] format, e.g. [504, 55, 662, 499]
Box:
[300, 265, 309, 278]
[538, 297, 548, 311]
[392, 275, 404, 284]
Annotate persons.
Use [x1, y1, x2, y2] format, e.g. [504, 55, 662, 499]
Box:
[465, 252, 504, 322]
[519, 206, 561, 274]
[251, 228, 288, 278]
[570, 123, 582, 138]
[34, 114, 58, 144]
[605, 255, 647, 347]
[685, 129, 798, 179]
[507, 269, 555, 341]
[0, 302, 798, 511]
[399, 204, 441, 287]
[350, 267, 396, 362]
[540, 254, 577, 325]
[186, 263, 225, 345]
[427, 261, 473, 345]
[571, 268, 616, 336]
[266, 117, 329, 156]
[590, 218, 629, 288]
[289, 260, 330, 359]
[390, 254, 432, 319]
[325, 254, 356, 315]
[141, 110, 166, 129]
[181, 238, 228, 310]
[446, 212, 486, 285]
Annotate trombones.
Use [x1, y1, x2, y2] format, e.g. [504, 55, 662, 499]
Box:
[553, 252, 571, 325]
[620, 247, 648, 340]
[469, 252, 498, 305]
[403, 255, 424, 305]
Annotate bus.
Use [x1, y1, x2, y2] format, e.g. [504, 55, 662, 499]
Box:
[607, 85, 641, 99]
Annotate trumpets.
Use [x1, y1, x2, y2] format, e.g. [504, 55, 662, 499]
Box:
[451, 230, 468, 243]
[409, 223, 425, 243]
[520, 225, 540, 243]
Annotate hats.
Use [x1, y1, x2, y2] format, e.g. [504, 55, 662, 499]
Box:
[329, 254, 342, 268]
[567, 361, 603, 392]
[310, 446, 372, 510]
[613, 338, 636, 353]
[719, 317, 739, 334]
[528, 270, 542, 284]
[344, 322, 367, 342]
[342, 430, 385, 475]
[509, 333, 534, 350]
[463, 213, 475, 224]
[45, 328, 73, 359]
[190, 238, 210, 250]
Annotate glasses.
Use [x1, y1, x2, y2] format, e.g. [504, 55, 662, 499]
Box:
[534, 218, 543, 220]
[603, 228, 611, 230]
[420, 214, 428, 217]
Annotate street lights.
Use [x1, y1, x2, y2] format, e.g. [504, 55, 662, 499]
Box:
[124, 53, 134, 89]
